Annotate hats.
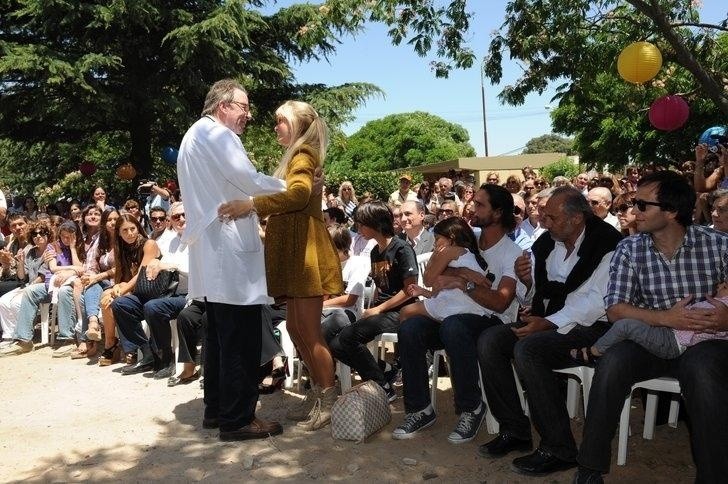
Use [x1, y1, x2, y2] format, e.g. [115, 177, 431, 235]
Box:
[399, 174, 414, 184]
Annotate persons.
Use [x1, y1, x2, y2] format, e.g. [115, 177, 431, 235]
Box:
[710, 188, 728, 232]
[125, 200, 146, 229]
[319, 200, 419, 404]
[167, 297, 209, 389]
[508, 192, 531, 253]
[98, 212, 163, 367]
[439, 200, 460, 221]
[0, 204, 108, 360]
[0, 220, 55, 348]
[56, 201, 63, 212]
[333, 183, 358, 217]
[439, 177, 459, 206]
[67, 204, 81, 219]
[486, 171, 500, 184]
[533, 179, 544, 190]
[515, 190, 549, 250]
[159, 201, 189, 274]
[522, 166, 533, 179]
[461, 203, 474, 222]
[506, 174, 522, 193]
[146, 206, 167, 240]
[694, 143, 728, 194]
[112, 202, 189, 380]
[621, 166, 640, 207]
[534, 186, 558, 230]
[614, 190, 637, 235]
[321, 226, 366, 341]
[396, 200, 435, 254]
[387, 176, 418, 209]
[569, 280, 727, 365]
[23, 196, 39, 220]
[465, 185, 475, 202]
[585, 186, 621, 233]
[46, 204, 59, 216]
[575, 173, 588, 192]
[220, 101, 343, 433]
[477, 185, 624, 476]
[392, 184, 525, 445]
[417, 183, 432, 213]
[526, 172, 536, 184]
[574, 171, 728, 483]
[92, 186, 109, 211]
[257, 211, 291, 395]
[71, 208, 123, 359]
[44, 220, 87, 335]
[0, 210, 31, 296]
[144, 178, 170, 210]
[392, 209, 407, 241]
[177, 79, 283, 443]
[399, 215, 495, 323]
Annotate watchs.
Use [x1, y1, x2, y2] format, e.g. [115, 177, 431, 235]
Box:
[463, 281, 476, 295]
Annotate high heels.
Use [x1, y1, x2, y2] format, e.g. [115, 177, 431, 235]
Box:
[258, 376, 285, 395]
[97, 336, 121, 367]
[85, 320, 102, 342]
[70, 340, 98, 359]
[124, 350, 136, 363]
[271, 355, 291, 379]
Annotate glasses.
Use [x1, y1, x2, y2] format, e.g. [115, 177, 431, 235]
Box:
[29, 229, 48, 237]
[229, 101, 251, 114]
[591, 199, 607, 207]
[630, 196, 662, 212]
[613, 203, 632, 213]
[125, 204, 137, 211]
[149, 215, 168, 223]
[437, 207, 455, 215]
[171, 210, 187, 220]
[342, 187, 352, 191]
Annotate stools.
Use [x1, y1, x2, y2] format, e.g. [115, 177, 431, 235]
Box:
[296, 346, 356, 395]
[525, 365, 596, 422]
[369, 332, 425, 362]
[137, 318, 181, 367]
[40, 302, 59, 345]
[428, 350, 527, 434]
[617, 376, 684, 467]
[273, 318, 295, 390]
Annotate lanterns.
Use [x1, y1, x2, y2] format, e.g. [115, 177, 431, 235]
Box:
[161, 147, 179, 164]
[163, 179, 177, 194]
[648, 95, 689, 132]
[698, 126, 725, 148]
[618, 42, 663, 85]
[116, 164, 136, 181]
[79, 160, 97, 178]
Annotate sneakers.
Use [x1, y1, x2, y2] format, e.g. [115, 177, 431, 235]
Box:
[285, 388, 317, 420]
[477, 429, 533, 459]
[391, 412, 438, 440]
[447, 399, 489, 445]
[52, 343, 77, 358]
[0, 341, 34, 358]
[510, 439, 579, 477]
[219, 417, 284, 441]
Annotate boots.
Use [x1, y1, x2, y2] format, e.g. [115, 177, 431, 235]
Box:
[297, 385, 340, 431]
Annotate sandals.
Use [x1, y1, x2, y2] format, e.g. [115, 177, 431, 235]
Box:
[566, 343, 600, 370]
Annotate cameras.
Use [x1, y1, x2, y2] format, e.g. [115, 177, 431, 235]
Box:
[138, 179, 151, 194]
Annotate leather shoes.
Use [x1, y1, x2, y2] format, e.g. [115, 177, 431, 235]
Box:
[167, 368, 201, 388]
[154, 363, 176, 379]
[120, 355, 162, 376]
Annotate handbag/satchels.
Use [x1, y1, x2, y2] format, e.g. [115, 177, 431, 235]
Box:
[329, 377, 393, 441]
[131, 264, 179, 302]
